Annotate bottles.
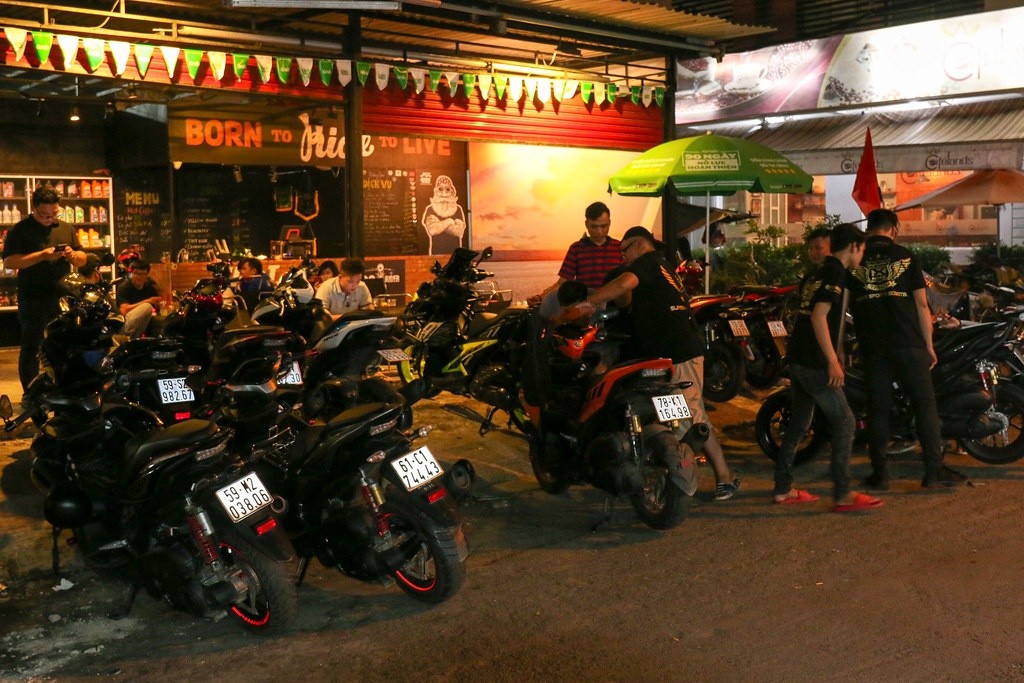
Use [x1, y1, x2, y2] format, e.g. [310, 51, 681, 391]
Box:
[24, 180, 109, 198]
[56, 205, 109, 223]
[77, 228, 103, 247]
[0, 230, 9, 252]
[0, 287, 20, 306]
[181, 250, 189, 262]
[0, 204, 21, 223]
[0, 181, 15, 197]
[0, 257, 19, 275]
[161, 252, 170, 263]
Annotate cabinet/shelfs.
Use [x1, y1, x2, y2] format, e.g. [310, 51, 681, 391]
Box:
[0, 173, 116, 310]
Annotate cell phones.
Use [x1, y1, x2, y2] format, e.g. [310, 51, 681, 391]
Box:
[54, 244, 67, 253]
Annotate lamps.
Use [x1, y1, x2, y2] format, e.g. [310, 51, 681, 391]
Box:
[554, 35, 583, 57]
[233, 164, 243, 183]
[69, 107, 81, 122]
[487, 20, 507, 35]
[268, 166, 278, 183]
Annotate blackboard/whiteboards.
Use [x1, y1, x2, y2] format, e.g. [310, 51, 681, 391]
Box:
[113, 190, 159, 262]
[180, 197, 218, 263]
[362, 168, 417, 256]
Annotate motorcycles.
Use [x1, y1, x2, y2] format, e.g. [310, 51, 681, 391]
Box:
[0, 245, 1024, 638]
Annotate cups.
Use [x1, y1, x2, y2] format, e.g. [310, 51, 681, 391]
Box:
[270, 240, 283, 260]
[372, 297, 397, 307]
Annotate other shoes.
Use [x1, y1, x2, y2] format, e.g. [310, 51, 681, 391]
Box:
[921, 469, 968, 488]
[711, 477, 740, 500]
[860, 477, 892, 492]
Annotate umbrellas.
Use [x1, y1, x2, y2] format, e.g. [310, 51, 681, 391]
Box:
[608, 131, 814, 295]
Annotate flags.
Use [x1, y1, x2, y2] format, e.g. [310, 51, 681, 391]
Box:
[852, 127, 881, 217]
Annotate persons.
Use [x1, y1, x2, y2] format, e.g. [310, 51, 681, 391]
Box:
[806, 228, 831, 264]
[555, 278, 608, 376]
[557, 202, 624, 292]
[3, 186, 86, 407]
[849, 207, 966, 489]
[117, 259, 162, 316]
[309, 261, 339, 292]
[568, 226, 741, 500]
[771, 223, 884, 511]
[79, 252, 153, 338]
[237, 257, 274, 304]
[316, 256, 375, 320]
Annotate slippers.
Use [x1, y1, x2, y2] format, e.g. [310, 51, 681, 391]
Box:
[777, 487, 821, 503]
[834, 493, 884, 511]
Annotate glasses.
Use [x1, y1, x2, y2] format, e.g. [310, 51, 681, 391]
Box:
[620, 239, 636, 259]
[35, 207, 58, 220]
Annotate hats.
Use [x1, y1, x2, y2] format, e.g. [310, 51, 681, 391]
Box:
[623, 227, 667, 250]
